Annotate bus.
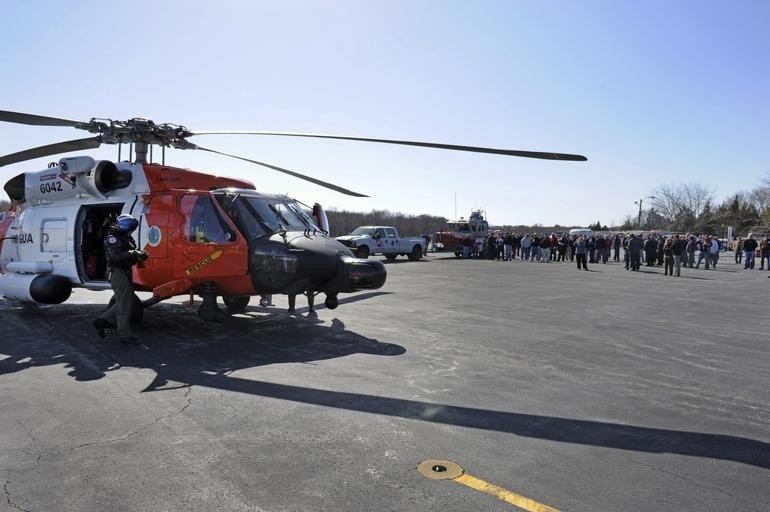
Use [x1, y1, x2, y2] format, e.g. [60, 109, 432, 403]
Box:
[569, 229, 592, 235]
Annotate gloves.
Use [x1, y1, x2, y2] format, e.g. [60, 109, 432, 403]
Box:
[129, 249, 150, 262]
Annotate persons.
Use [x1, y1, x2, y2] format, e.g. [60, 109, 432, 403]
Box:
[287, 288, 315, 314]
[91, 213, 151, 346]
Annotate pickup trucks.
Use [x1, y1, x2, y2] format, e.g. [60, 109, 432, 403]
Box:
[334, 225, 427, 261]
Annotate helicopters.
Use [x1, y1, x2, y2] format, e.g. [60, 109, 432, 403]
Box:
[0, 109, 588, 324]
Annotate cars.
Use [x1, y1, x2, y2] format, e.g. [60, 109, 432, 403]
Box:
[423, 235, 445, 252]
[551, 231, 569, 237]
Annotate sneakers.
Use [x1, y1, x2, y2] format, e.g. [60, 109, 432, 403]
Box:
[119, 335, 144, 345]
[624, 260, 770, 277]
[500, 257, 620, 271]
[288, 306, 316, 313]
[92, 318, 106, 339]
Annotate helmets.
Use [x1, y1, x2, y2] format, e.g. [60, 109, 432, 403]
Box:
[116, 214, 138, 235]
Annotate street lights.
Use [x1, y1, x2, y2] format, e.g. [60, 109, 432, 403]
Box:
[649, 196, 655, 225]
[721, 223, 724, 246]
[634, 199, 642, 226]
